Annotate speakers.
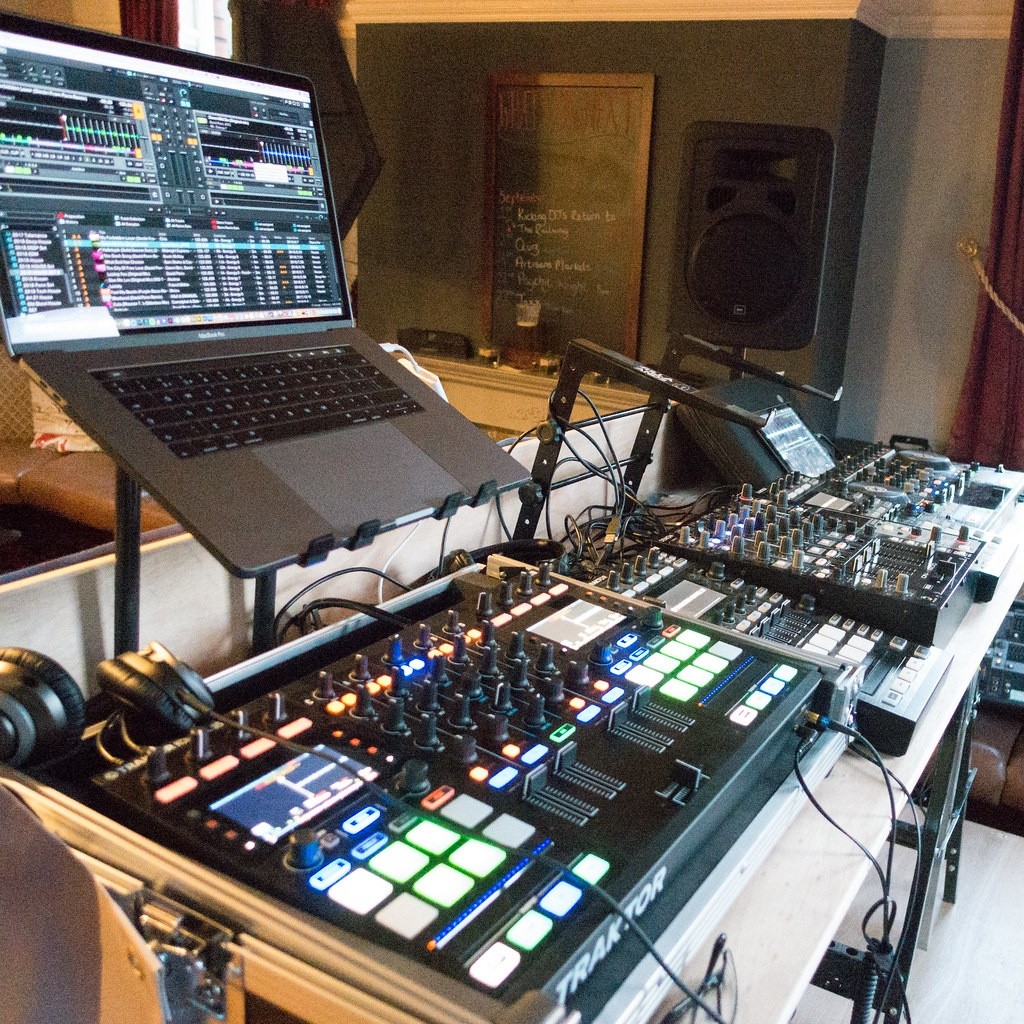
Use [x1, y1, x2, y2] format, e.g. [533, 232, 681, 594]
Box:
[670, 120, 836, 349]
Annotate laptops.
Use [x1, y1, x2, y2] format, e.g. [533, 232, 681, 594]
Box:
[0, 9, 533, 579]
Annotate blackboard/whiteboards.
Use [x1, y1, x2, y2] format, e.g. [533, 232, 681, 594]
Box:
[479, 72, 659, 380]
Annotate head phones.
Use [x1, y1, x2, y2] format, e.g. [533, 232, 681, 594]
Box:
[0, 643, 217, 772]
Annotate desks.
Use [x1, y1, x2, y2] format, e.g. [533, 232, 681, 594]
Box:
[0, 463, 1024, 1024]
